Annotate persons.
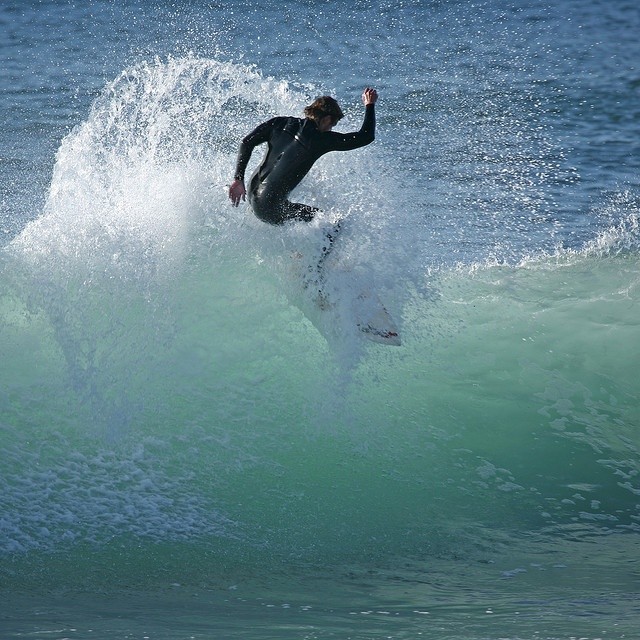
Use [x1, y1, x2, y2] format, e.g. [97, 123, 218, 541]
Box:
[228, 88, 378, 224]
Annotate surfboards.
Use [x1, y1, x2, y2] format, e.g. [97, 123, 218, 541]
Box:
[255, 252, 401, 346]
